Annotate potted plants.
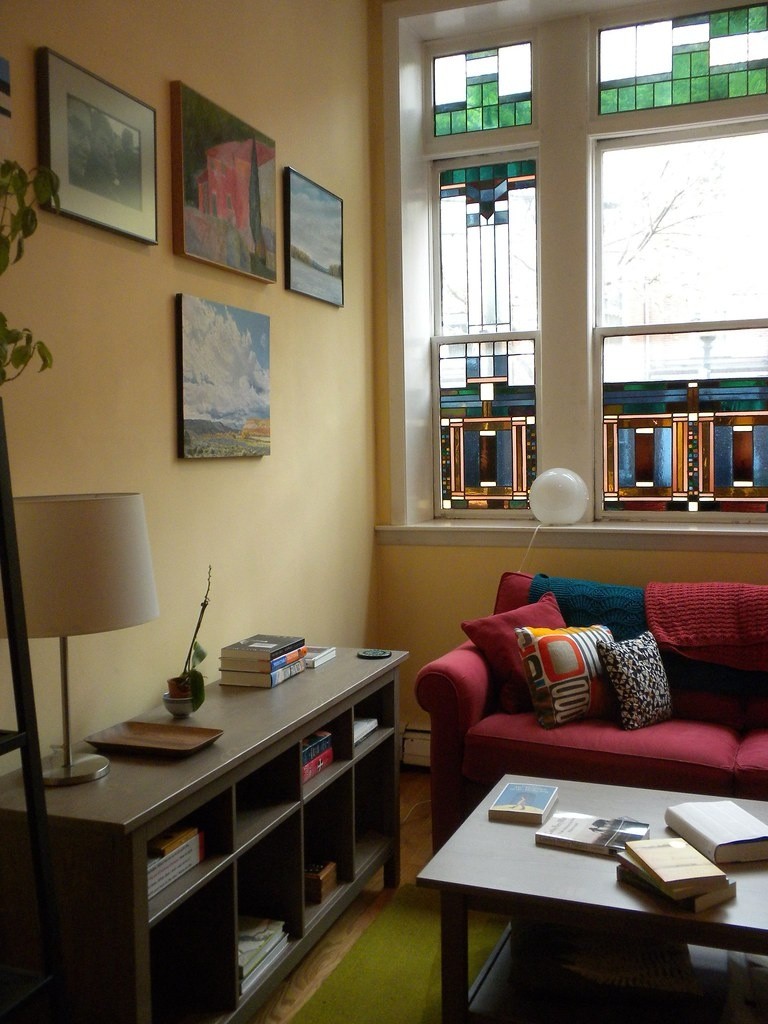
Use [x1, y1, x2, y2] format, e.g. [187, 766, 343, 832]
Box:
[161, 563, 212, 719]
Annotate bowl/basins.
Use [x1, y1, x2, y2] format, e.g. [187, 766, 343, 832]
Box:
[162, 693, 193, 719]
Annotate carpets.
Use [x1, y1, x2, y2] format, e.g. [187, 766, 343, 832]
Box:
[287, 883, 753, 1024]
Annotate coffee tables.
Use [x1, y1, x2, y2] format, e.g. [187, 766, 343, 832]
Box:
[416, 774, 768, 1024]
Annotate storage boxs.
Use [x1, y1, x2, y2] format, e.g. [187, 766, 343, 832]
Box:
[304, 861, 338, 905]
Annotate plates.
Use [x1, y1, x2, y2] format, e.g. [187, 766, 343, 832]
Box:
[83, 721, 225, 755]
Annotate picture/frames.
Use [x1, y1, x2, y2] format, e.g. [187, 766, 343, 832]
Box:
[34, 46, 160, 246]
[174, 292, 271, 460]
[282, 166, 346, 309]
[169, 79, 278, 286]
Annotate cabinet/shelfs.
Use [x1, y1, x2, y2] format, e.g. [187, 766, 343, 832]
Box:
[0, 647, 409, 1024]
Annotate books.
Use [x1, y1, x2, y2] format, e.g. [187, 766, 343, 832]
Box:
[147, 826, 204, 901]
[535, 810, 650, 857]
[665, 800, 768, 863]
[302, 730, 334, 785]
[353, 718, 379, 749]
[488, 782, 559, 825]
[617, 837, 736, 913]
[218, 634, 337, 688]
[237, 917, 290, 995]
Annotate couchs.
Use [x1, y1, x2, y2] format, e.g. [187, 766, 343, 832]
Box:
[414, 570, 768, 858]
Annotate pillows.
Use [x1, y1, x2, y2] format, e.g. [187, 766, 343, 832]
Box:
[459, 590, 569, 715]
[597, 631, 674, 732]
[514, 622, 616, 731]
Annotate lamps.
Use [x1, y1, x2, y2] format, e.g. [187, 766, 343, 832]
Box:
[516, 467, 589, 572]
[0, 491, 159, 791]
